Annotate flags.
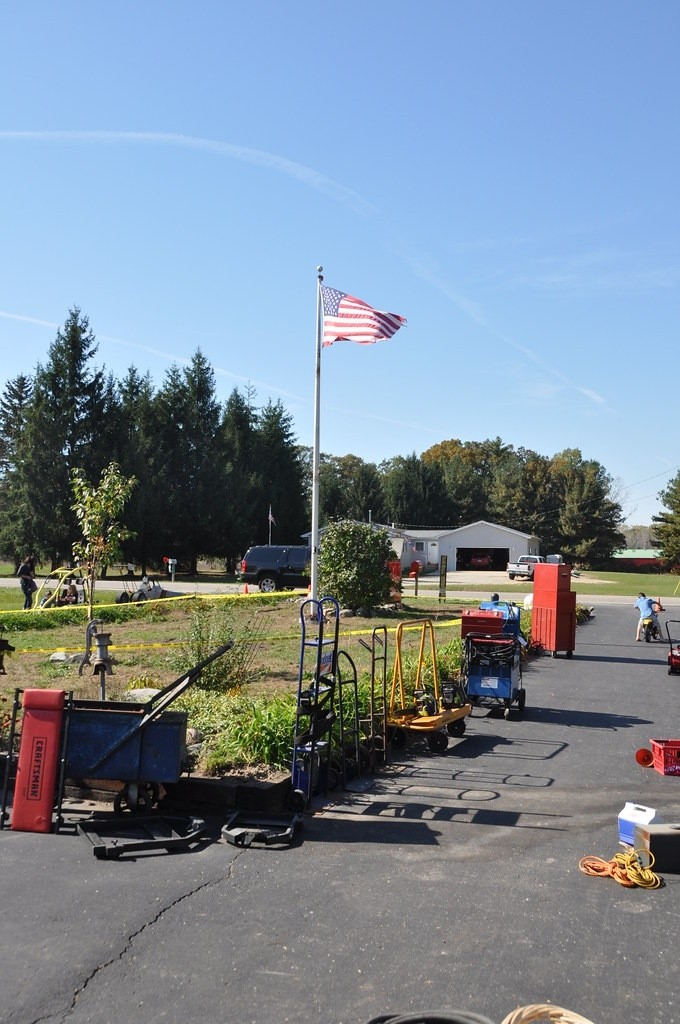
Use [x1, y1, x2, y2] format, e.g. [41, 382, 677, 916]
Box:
[320, 283, 407, 346]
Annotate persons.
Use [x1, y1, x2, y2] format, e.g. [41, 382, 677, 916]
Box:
[17, 556, 37, 610]
[634, 592, 666, 641]
[40, 591, 57, 609]
[60, 585, 78, 606]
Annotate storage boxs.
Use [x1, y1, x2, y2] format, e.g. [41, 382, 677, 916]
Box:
[617, 801, 680, 875]
[650, 739, 680, 775]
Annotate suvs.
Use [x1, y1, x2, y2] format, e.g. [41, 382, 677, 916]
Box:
[237, 545, 311, 593]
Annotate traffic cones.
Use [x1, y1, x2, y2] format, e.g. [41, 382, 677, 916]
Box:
[656, 599, 661, 611]
[244, 585, 248, 594]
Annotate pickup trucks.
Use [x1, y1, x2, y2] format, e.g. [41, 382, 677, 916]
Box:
[506, 555, 550, 580]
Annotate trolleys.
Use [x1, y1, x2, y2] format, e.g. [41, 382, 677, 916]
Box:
[288, 596, 475, 817]
[57, 642, 232, 812]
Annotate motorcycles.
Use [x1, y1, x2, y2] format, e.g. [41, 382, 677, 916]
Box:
[641, 608, 666, 643]
